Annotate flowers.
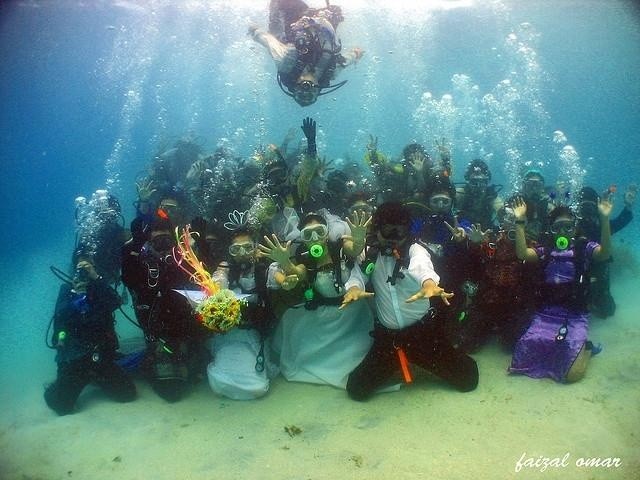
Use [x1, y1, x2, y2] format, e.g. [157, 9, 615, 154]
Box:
[167, 223, 249, 333]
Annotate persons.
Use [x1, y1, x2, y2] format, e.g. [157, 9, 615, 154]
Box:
[41, 116, 640, 416]
[247, 0, 365, 108]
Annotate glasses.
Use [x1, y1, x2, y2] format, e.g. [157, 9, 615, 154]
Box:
[430, 194, 453, 208]
[153, 235, 176, 251]
[379, 223, 410, 240]
[300, 224, 328, 242]
[158, 203, 178, 214]
[550, 221, 574, 235]
[349, 204, 373, 216]
[523, 178, 544, 188]
[267, 168, 285, 184]
[228, 242, 255, 257]
